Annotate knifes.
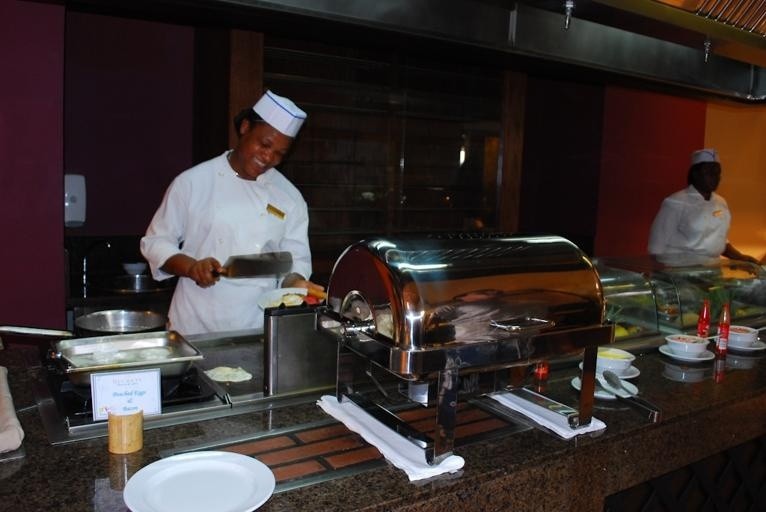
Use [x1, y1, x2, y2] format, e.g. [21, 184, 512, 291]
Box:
[197, 251, 292, 277]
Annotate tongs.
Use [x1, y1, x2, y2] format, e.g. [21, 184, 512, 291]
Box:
[594, 371, 662, 423]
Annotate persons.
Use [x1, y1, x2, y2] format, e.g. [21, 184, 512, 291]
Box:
[646, 148, 759, 269]
[138, 87, 314, 343]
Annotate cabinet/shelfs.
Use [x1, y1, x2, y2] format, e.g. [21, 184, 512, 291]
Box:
[230, 27, 528, 278]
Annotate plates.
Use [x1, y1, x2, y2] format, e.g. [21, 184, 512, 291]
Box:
[579, 361, 640, 379]
[122, 450, 275, 512]
[658, 344, 715, 363]
[571, 376, 638, 400]
[714, 338, 766, 353]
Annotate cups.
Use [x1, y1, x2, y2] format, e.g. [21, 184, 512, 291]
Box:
[108, 405, 143, 455]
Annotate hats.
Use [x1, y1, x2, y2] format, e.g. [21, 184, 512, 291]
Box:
[252, 89, 307, 138]
[690, 149, 721, 165]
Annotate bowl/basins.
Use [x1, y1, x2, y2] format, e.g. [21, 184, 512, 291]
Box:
[122, 263, 146, 274]
[75, 309, 167, 337]
[595, 347, 636, 374]
[98, 269, 176, 293]
[657, 304, 689, 322]
[258, 287, 324, 309]
[717, 325, 758, 347]
[665, 335, 710, 357]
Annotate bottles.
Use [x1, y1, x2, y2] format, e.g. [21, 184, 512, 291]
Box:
[716, 304, 730, 355]
[696, 297, 711, 338]
